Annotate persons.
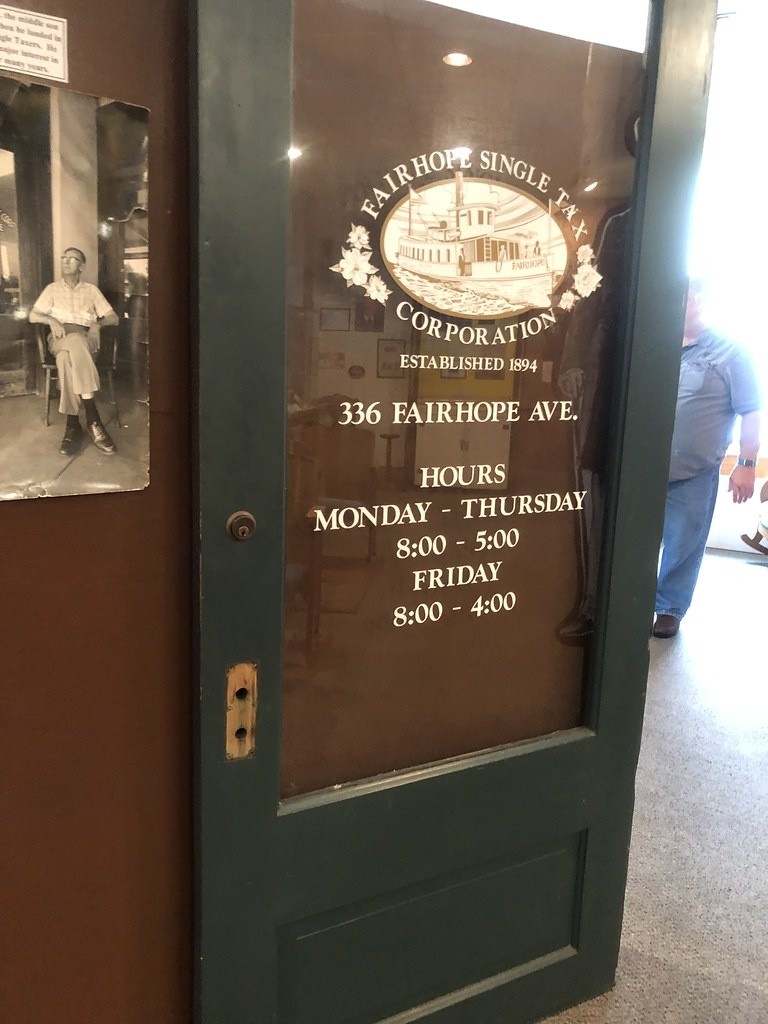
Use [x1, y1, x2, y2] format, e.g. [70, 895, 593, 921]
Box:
[29, 248, 119, 457]
[653, 274, 762, 637]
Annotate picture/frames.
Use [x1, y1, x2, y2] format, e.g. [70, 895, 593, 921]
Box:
[376, 338, 405, 378]
[319, 307, 350, 331]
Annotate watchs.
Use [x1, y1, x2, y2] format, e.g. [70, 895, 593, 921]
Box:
[738, 459, 756, 467]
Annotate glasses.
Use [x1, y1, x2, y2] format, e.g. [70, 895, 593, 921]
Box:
[61, 256, 82, 263]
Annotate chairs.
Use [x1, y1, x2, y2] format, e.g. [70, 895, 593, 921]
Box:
[33, 321, 122, 428]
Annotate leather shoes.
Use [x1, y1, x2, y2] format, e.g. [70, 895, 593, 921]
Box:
[87, 420, 116, 455]
[652, 613, 680, 637]
[60, 424, 83, 456]
